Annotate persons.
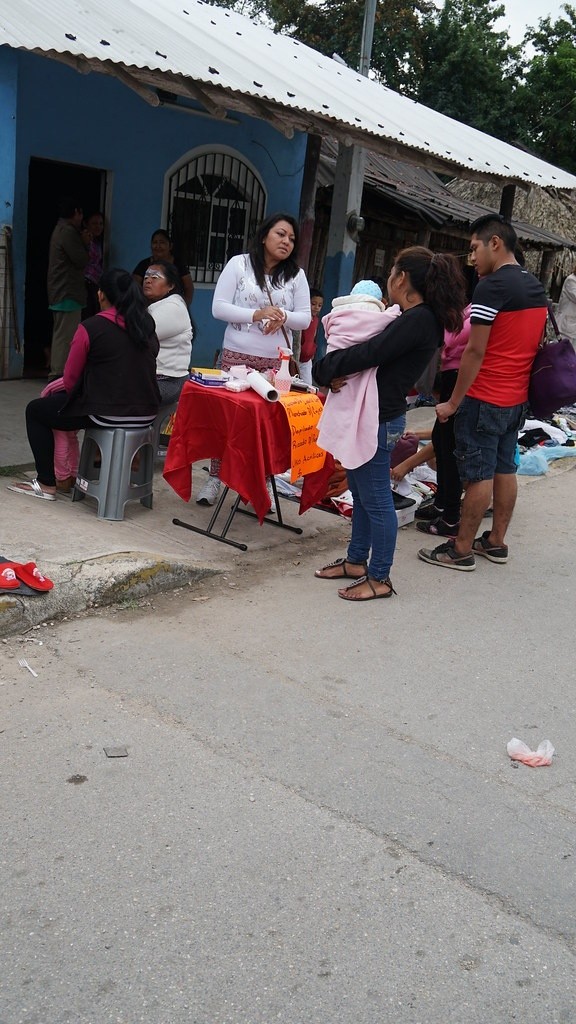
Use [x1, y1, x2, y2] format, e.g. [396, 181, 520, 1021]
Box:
[196, 212, 311, 512]
[141, 264, 194, 404]
[415, 268, 478, 536]
[313, 246, 466, 601]
[81, 210, 106, 316]
[420, 213, 549, 572]
[132, 228, 192, 311]
[41, 374, 82, 490]
[555, 266, 576, 352]
[47, 202, 91, 381]
[8, 270, 160, 500]
[300, 288, 323, 385]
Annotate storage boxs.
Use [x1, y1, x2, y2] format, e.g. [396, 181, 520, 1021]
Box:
[395, 504, 417, 528]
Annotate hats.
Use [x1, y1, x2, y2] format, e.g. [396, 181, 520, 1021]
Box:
[350, 280, 382, 302]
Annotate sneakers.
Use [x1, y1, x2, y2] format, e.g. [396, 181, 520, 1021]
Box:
[196, 480, 223, 505]
[266, 479, 276, 513]
[416, 516, 461, 539]
[417, 538, 476, 571]
[415, 502, 447, 521]
[470, 531, 508, 564]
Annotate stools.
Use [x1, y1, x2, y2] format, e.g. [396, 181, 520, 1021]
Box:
[154, 401, 178, 457]
[72, 426, 156, 521]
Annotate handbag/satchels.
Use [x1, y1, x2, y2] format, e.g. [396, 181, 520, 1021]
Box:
[289, 359, 301, 379]
[525, 301, 576, 420]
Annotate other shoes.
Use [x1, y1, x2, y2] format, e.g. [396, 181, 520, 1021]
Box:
[55, 476, 72, 492]
[460, 500, 496, 518]
[71, 475, 78, 488]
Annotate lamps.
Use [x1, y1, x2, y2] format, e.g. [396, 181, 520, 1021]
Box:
[348, 214, 365, 233]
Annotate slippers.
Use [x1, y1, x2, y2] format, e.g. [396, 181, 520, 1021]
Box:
[8, 478, 56, 501]
[8, 561, 54, 590]
[0, 561, 20, 588]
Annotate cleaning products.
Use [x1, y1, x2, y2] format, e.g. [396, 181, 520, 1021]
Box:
[275, 346, 294, 395]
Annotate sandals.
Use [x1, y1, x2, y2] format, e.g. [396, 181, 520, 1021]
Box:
[338, 575, 397, 601]
[312, 557, 369, 580]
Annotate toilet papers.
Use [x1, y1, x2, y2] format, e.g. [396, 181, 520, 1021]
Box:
[246, 372, 281, 403]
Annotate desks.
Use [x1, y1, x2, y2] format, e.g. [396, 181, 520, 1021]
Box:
[162, 381, 337, 551]
